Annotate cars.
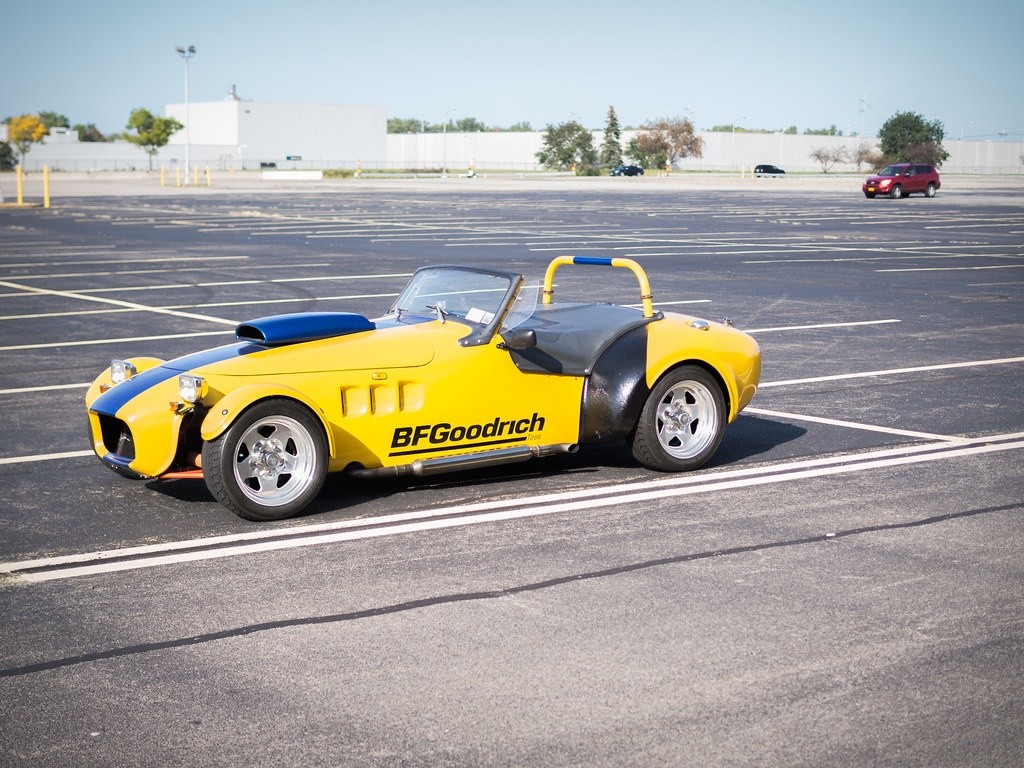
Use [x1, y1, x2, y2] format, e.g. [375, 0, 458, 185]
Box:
[609, 166, 645, 177]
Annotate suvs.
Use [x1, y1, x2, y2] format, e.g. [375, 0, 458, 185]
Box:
[755, 164, 785, 178]
[862, 163, 941, 200]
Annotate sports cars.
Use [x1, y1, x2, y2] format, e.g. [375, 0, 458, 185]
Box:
[81, 255, 763, 522]
